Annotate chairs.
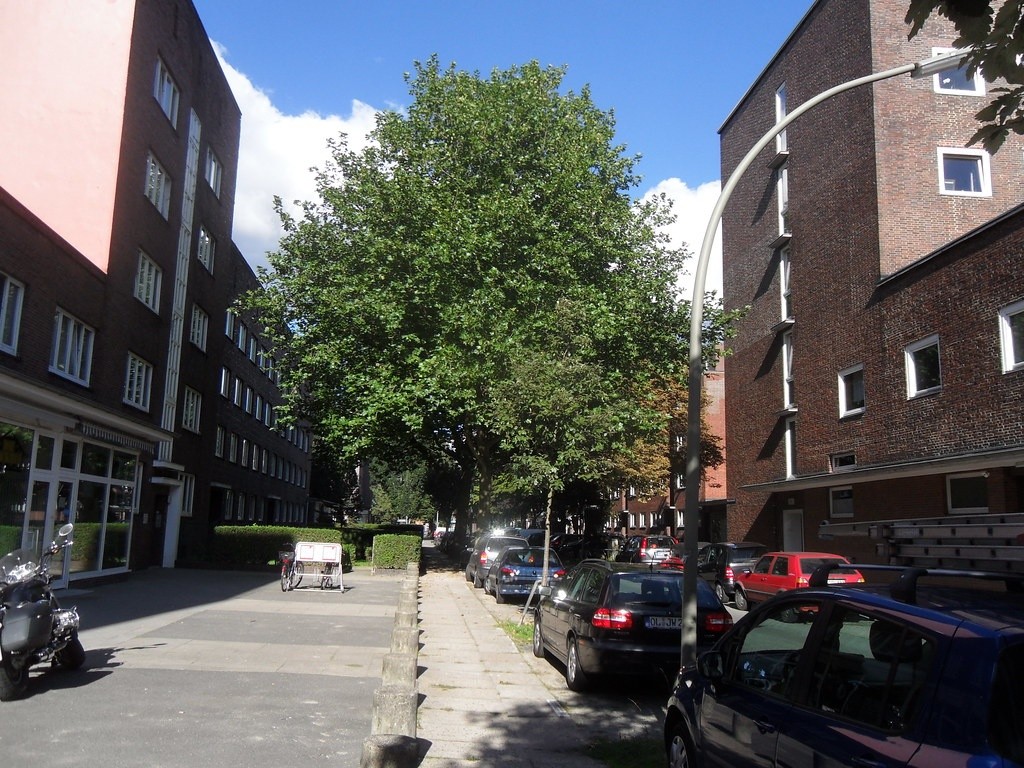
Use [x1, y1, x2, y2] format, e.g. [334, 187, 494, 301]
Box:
[532, 552, 545, 564]
[841, 620, 933, 728]
[641, 580, 667, 602]
[506, 553, 522, 563]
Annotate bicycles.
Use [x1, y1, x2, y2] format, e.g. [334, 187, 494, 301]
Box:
[320, 562, 334, 590]
[279, 543, 304, 592]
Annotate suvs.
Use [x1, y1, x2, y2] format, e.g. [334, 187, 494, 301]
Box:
[533, 558, 734, 692]
[444, 527, 768, 604]
[663, 562, 1024, 768]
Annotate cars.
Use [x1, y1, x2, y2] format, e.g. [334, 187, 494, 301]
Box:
[734, 551, 865, 623]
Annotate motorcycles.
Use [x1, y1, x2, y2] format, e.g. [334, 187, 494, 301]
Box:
[0, 523, 86, 701]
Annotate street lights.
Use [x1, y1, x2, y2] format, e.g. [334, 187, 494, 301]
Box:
[679, 47, 980, 669]
